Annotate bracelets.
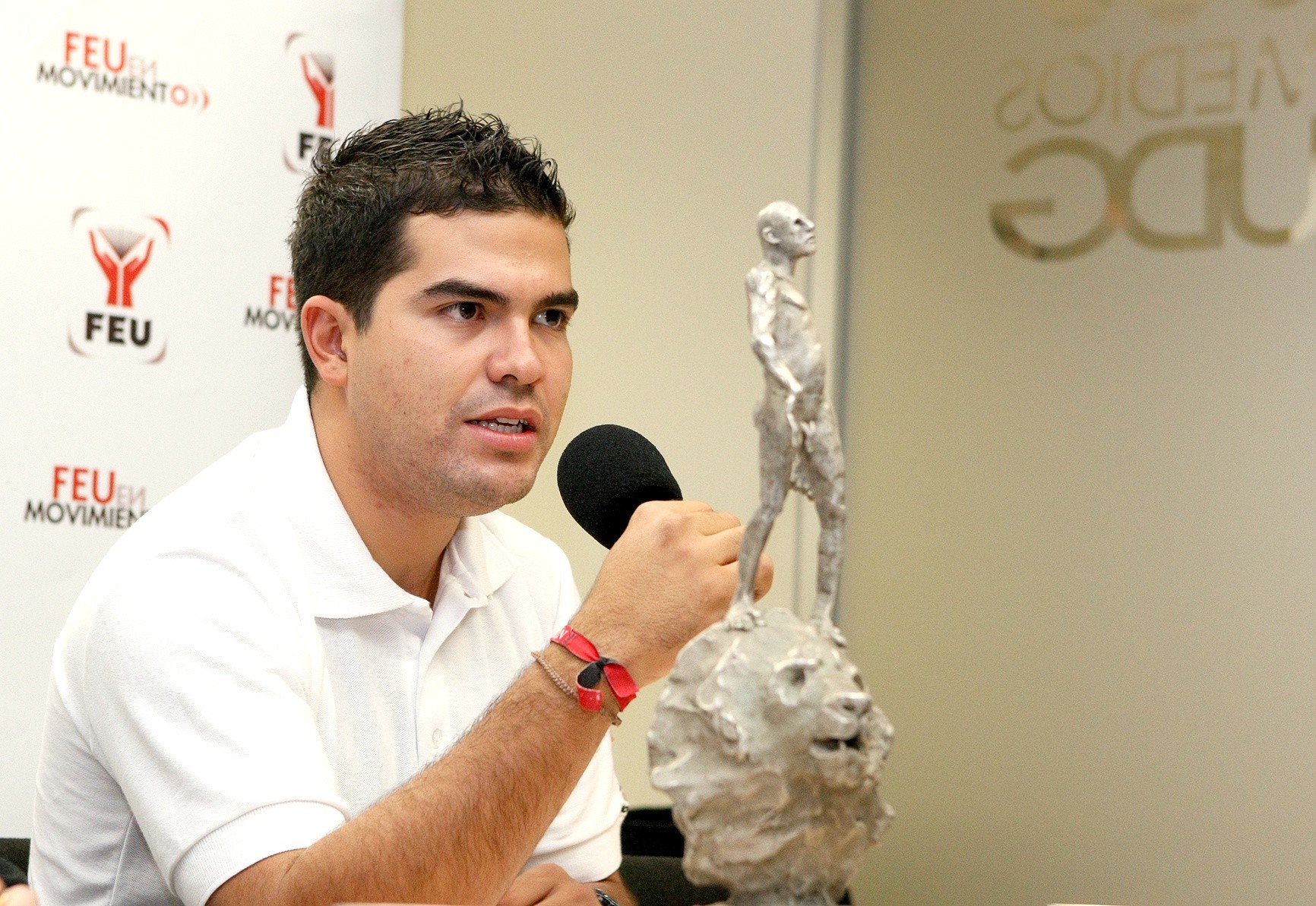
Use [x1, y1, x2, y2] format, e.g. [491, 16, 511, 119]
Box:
[594, 889, 619, 906]
[550, 624, 640, 712]
[530, 650, 622, 727]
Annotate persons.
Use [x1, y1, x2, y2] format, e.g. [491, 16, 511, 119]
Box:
[0, 858, 37, 906]
[724, 200, 852, 647]
[28, 95, 773, 906]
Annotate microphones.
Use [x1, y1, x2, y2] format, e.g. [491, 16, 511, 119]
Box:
[556, 425, 681, 549]
